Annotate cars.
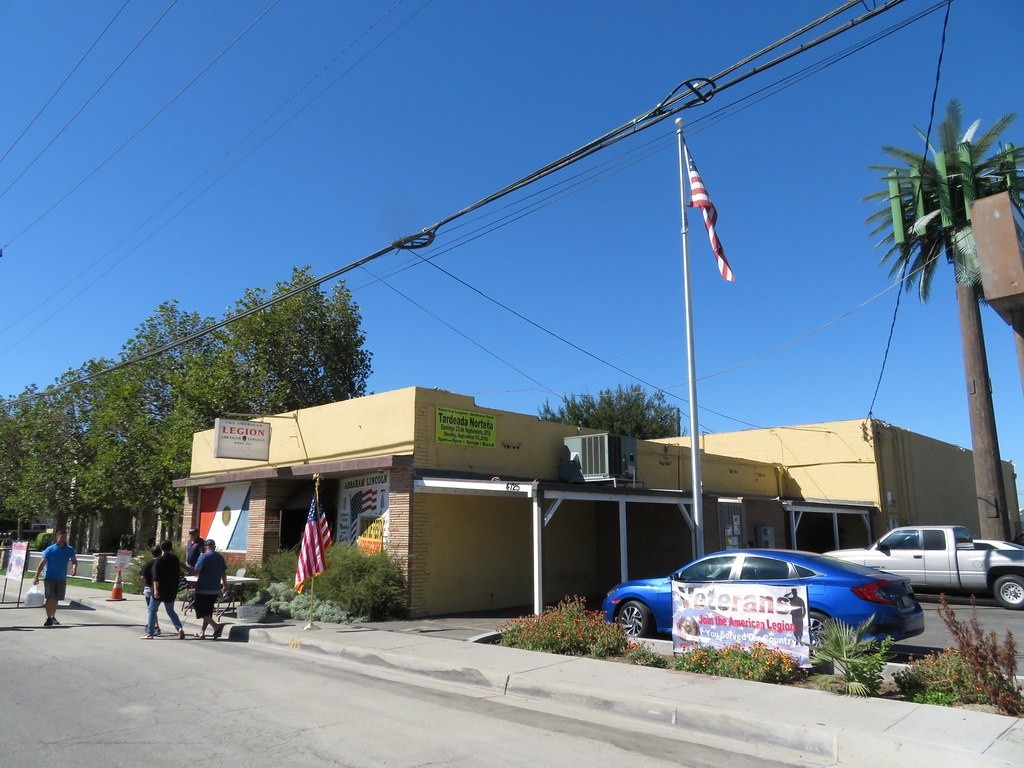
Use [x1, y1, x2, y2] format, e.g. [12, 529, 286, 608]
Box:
[972, 538, 1024, 550]
[601, 547, 926, 650]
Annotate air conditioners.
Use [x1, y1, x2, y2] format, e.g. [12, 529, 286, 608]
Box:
[559, 431, 637, 479]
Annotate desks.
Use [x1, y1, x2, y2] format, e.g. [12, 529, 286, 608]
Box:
[182, 575, 260, 617]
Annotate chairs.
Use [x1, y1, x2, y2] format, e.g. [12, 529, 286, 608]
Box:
[216, 568, 247, 615]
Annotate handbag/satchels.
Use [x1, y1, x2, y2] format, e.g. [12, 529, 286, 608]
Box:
[177, 571, 189, 592]
[25, 583, 45, 608]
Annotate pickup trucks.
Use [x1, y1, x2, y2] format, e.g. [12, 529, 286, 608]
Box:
[819, 524, 1024, 611]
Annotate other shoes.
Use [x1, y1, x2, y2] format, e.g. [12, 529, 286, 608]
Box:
[145, 625, 149, 632]
[153, 626, 160, 636]
[52, 617, 59, 624]
[178, 627, 185, 639]
[193, 631, 206, 638]
[44, 618, 53, 626]
[139, 633, 153, 639]
[212, 625, 223, 640]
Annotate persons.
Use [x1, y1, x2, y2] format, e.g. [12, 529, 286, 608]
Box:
[194, 540, 228, 639]
[33, 530, 78, 626]
[184, 529, 206, 609]
[140, 540, 185, 640]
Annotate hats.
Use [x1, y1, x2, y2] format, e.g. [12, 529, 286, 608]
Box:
[188, 527, 199, 535]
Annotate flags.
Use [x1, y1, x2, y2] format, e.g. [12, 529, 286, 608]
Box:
[682, 139, 735, 282]
[294, 493, 333, 594]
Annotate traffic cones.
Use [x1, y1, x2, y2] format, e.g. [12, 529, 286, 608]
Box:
[106, 566, 126, 602]
